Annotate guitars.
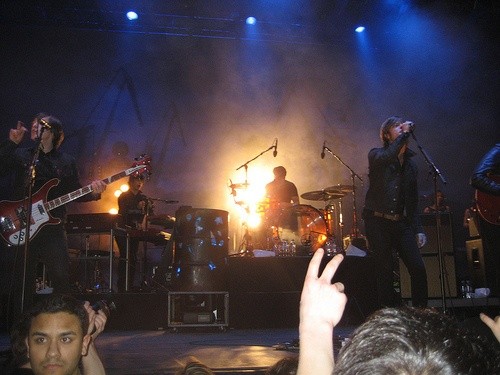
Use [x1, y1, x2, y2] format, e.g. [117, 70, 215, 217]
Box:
[0, 153, 153, 248]
[472, 173, 500, 225]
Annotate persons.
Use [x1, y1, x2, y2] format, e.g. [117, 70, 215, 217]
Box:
[424, 191, 450, 214]
[0, 293, 112, 375]
[0, 112, 106, 335]
[360, 116, 428, 310]
[469, 140, 500, 300]
[166, 249, 500, 374]
[114, 172, 173, 286]
[260, 167, 301, 254]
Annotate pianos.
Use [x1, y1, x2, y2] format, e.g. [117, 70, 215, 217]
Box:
[63, 212, 172, 293]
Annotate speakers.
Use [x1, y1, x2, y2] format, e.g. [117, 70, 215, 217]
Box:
[465, 239, 485, 267]
[398, 254, 457, 297]
[413, 212, 453, 254]
[135, 296, 166, 330]
[92, 291, 139, 331]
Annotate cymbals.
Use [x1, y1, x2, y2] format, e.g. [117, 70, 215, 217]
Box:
[300, 191, 345, 201]
[228, 183, 251, 189]
[323, 185, 358, 195]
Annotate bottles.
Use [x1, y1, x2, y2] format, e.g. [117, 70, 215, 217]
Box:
[465, 280, 473, 298]
[274, 238, 296, 256]
[460, 280, 466, 298]
[326, 236, 338, 256]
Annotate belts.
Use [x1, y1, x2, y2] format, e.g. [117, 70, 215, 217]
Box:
[374, 211, 400, 222]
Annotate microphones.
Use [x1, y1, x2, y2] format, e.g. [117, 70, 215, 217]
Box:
[409, 123, 415, 128]
[321, 139, 326, 157]
[273, 141, 278, 157]
[38, 118, 55, 131]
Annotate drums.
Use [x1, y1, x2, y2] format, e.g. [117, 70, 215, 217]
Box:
[318, 208, 335, 235]
[269, 203, 330, 256]
